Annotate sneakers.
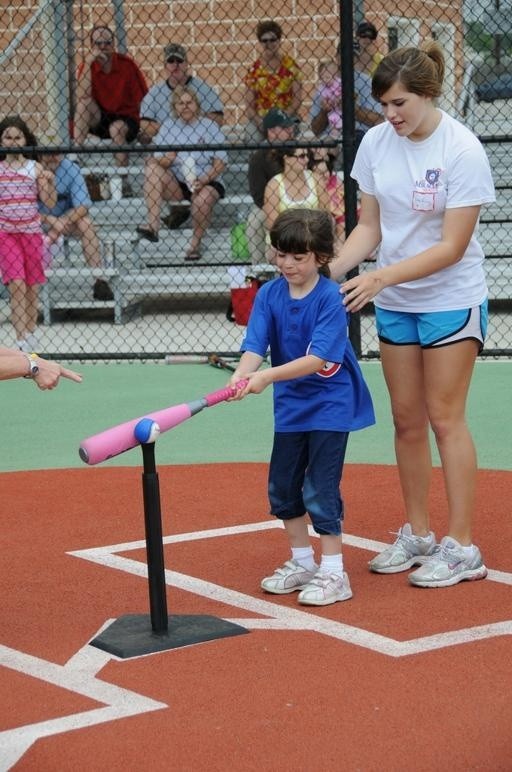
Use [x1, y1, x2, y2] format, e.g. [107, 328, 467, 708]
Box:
[260, 557, 323, 595]
[93, 278, 115, 302]
[407, 535, 489, 590]
[297, 567, 354, 607]
[368, 521, 440, 575]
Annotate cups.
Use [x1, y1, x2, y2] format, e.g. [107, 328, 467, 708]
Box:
[84, 173, 123, 202]
[103, 239, 116, 271]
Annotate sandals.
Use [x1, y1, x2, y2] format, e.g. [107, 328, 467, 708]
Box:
[185, 251, 202, 261]
[135, 222, 159, 243]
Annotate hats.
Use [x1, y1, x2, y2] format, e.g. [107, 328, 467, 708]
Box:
[356, 22, 378, 36]
[164, 43, 187, 61]
[263, 107, 302, 129]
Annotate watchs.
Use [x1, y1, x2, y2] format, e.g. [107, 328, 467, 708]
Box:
[24, 354, 39, 380]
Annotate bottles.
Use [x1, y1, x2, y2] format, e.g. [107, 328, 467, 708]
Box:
[229, 212, 250, 259]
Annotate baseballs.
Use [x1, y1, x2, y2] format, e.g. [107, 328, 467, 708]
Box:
[134, 418, 160, 444]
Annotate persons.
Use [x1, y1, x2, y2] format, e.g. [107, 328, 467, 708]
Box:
[136, 86, 227, 260]
[1, 116, 56, 349]
[308, 23, 391, 171]
[139, 44, 226, 133]
[37, 135, 115, 302]
[241, 20, 303, 146]
[228, 208, 376, 604]
[246, 110, 376, 316]
[0, 349, 82, 391]
[69, 27, 147, 199]
[322, 40, 496, 587]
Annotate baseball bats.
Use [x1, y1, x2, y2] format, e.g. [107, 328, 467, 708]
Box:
[78, 379, 248, 465]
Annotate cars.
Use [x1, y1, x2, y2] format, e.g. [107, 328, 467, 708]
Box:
[472, 70, 511, 106]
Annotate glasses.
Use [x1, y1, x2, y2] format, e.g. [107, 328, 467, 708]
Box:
[293, 153, 310, 159]
[261, 37, 280, 44]
[166, 57, 184, 64]
[360, 35, 377, 40]
[94, 38, 113, 45]
